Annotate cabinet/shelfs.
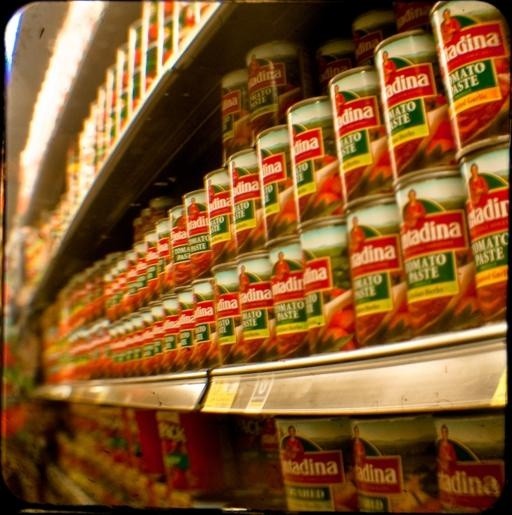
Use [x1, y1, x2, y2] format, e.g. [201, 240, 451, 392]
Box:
[0, 0, 512, 515]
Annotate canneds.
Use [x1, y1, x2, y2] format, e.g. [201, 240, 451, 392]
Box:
[21, 1, 511, 515]
